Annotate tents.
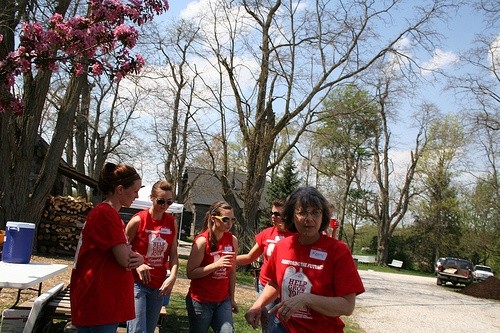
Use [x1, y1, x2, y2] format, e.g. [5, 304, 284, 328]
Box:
[121, 196, 184, 249]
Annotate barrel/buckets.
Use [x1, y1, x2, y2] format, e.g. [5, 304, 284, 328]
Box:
[161, 270, 171, 305]
[2, 221, 35, 264]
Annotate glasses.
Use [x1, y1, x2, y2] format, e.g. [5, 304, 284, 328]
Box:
[269, 212, 282, 217]
[214, 215, 236, 224]
[155, 198, 174, 206]
[296, 210, 323, 217]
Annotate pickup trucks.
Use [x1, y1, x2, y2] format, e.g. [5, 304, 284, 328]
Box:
[435, 257, 474, 286]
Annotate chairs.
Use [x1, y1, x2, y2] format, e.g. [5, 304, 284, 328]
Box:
[22, 283, 65, 333]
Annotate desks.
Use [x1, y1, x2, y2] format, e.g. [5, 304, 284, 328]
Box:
[0, 261, 69, 318]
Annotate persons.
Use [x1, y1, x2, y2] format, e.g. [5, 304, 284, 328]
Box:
[124, 181, 179, 333]
[70, 162, 144, 333]
[235, 200, 296, 333]
[186, 201, 239, 333]
[245, 186, 365, 333]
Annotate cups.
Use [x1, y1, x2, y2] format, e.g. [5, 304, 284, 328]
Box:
[222, 252, 236, 268]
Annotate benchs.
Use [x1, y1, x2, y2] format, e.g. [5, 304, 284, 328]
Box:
[388, 259, 403, 271]
[352, 254, 376, 266]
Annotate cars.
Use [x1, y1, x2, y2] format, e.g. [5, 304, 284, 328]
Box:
[472, 265, 495, 281]
[435, 258, 446, 276]
